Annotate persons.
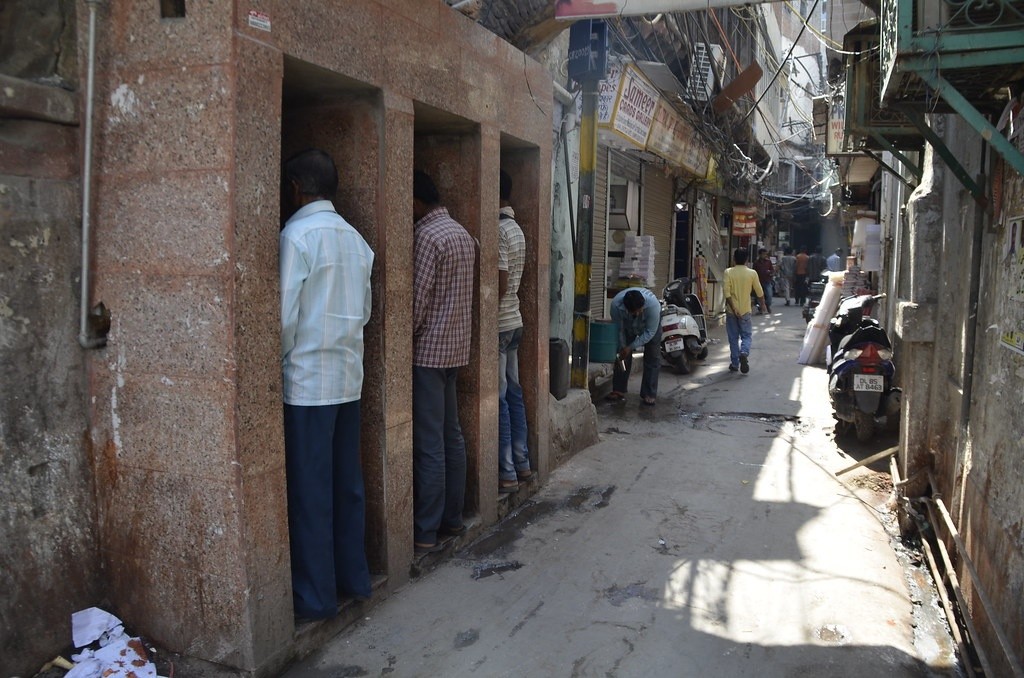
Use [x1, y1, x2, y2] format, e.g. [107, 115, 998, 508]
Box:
[278, 147, 376, 621]
[498, 169, 534, 496]
[779, 244, 843, 306]
[721, 248, 767, 374]
[751, 249, 774, 315]
[412, 170, 477, 553]
[607, 287, 661, 404]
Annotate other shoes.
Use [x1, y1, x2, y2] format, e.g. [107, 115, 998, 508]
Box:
[739, 352, 750, 375]
[728, 362, 736, 372]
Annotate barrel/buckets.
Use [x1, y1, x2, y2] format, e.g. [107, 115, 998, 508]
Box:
[549, 336, 570, 401]
[590, 321, 617, 363]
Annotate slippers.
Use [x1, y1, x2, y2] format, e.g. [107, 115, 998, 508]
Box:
[643, 393, 655, 403]
[294, 611, 316, 623]
[498, 477, 520, 493]
[441, 521, 468, 537]
[412, 539, 443, 554]
[340, 583, 370, 603]
[519, 472, 534, 481]
[606, 391, 624, 401]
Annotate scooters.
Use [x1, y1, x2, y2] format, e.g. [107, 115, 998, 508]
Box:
[829, 291, 903, 444]
[801, 275, 828, 324]
[655, 275, 712, 376]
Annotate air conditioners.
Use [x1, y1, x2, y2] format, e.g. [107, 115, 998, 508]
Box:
[685, 43, 727, 100]
